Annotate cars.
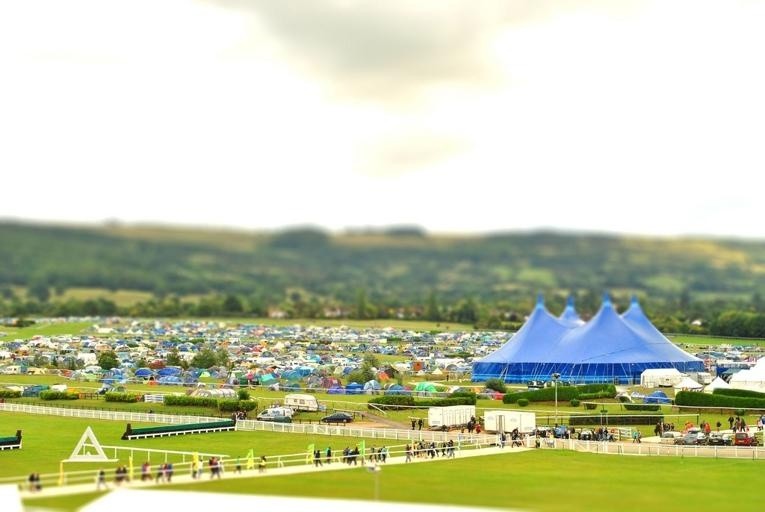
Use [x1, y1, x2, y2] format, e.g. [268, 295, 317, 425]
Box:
[321, 412, 354, 423]
[661, 428, 752, 446]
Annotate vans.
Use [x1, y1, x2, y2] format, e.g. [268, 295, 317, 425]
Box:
[256, 407, 292, 422]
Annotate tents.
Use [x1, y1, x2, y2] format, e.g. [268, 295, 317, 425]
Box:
[0, 313, 765, 400]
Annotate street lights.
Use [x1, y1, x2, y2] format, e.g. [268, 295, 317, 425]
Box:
[552, 373, 560, 428]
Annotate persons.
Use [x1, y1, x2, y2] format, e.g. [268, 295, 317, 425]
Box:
[235, 455, 241, 474]
[468, 415, 485, 434]
[757, 416, 765, 430]
[258, 454, 267, 474]
[685, 420, 711, 437]
[95, 461, 174, 490]
[716, 420, 722, 432]
[632, 430, 641, 444]
[728, 415, 746, 434]
[29, 473, 41, 493]
[501, 428, 520, 448]
[411, 418, 423, 431]
[655, 422, 675, 437]
[315, 446, 360, 466]
[192, 456, 221, 481]
[369, 444, 387, 463]
[406, 439, 455, 463]
[537, 424, 615, 443]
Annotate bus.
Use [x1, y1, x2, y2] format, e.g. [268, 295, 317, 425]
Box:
[284, 394, 318, 411]
